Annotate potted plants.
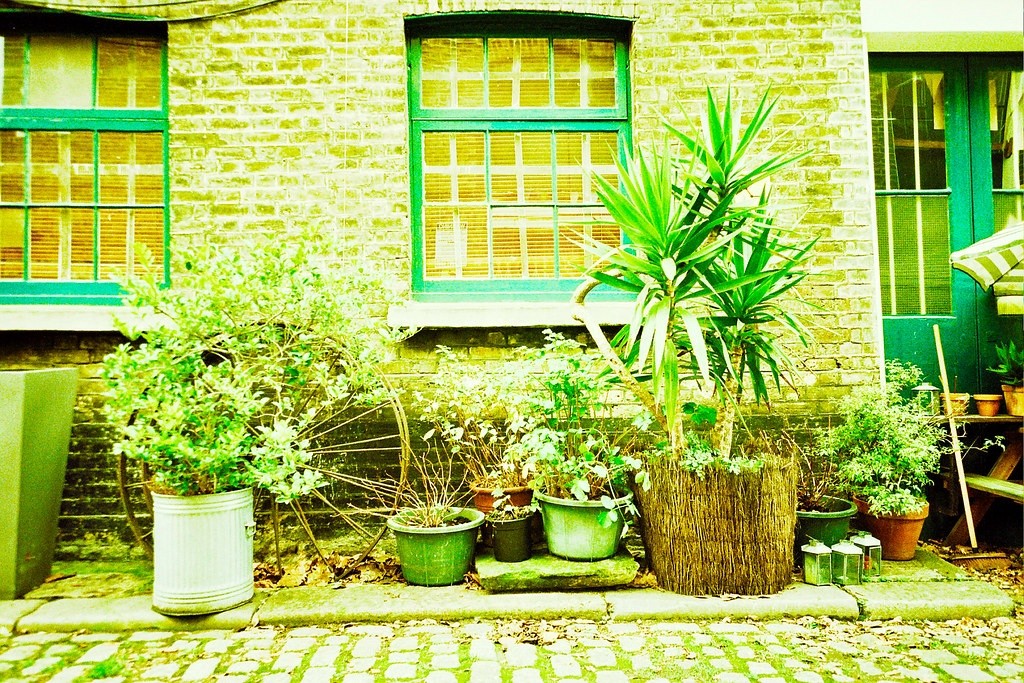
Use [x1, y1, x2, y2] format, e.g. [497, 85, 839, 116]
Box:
[93, 84, 1024, 615]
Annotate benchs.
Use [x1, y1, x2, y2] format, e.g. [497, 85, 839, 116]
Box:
[964, 471, 1024, 502]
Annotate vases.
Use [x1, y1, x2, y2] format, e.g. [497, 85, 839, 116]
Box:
[973, 393, 1002, 417]
[1, 367, 77, 600]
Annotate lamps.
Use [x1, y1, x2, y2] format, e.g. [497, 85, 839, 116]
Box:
[911, 382, 941, 417]
[850, 531, 882, 576]
[831, 539, 864, 586]
[800, 533, 832, 586]
